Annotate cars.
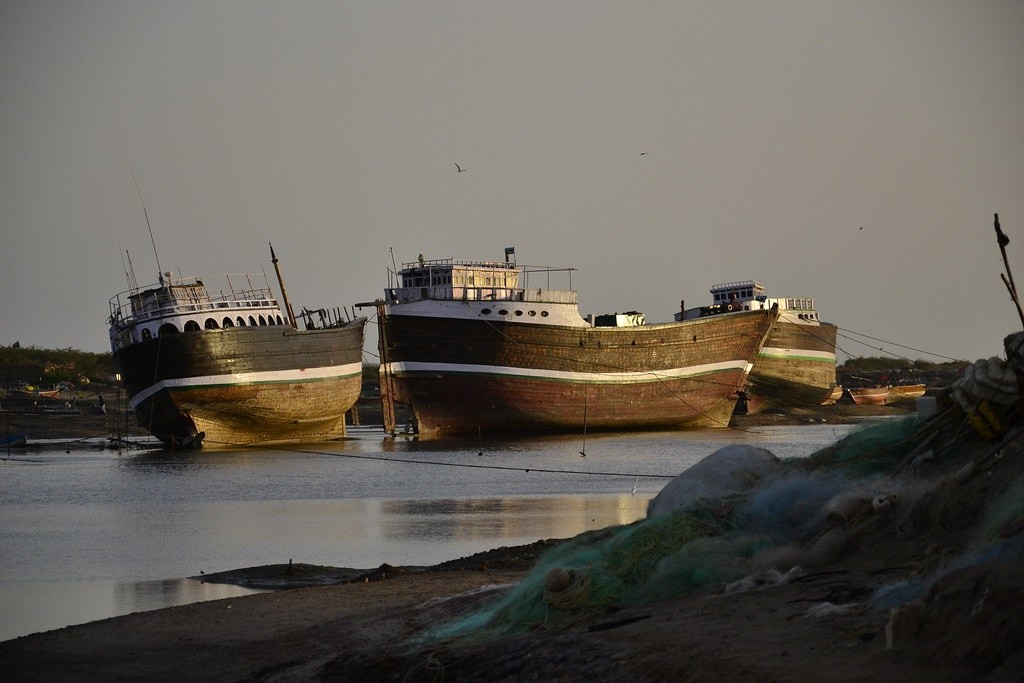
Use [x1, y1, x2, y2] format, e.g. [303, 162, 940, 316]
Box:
[56, 381, 77, 392]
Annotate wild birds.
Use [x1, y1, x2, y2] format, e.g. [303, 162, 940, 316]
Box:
[454, 161, 468, 175]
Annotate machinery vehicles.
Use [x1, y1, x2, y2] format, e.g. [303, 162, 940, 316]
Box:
[40, 364, 90, 389]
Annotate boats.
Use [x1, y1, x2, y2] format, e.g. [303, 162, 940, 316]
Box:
[7, 389, 61, 400]
[0, 432, 28, 450]
[672, 279, 842, 415]
[379, 245, 781, 431]
[847, 384, 926, 406]
[103, 161, 368, 454]
